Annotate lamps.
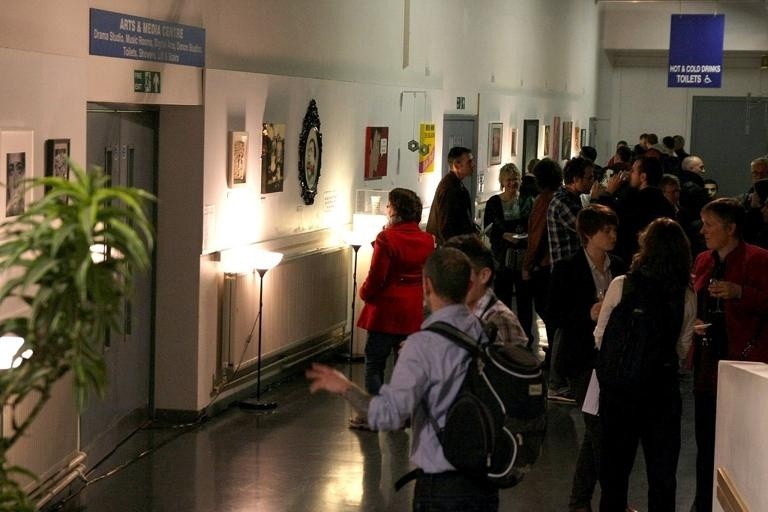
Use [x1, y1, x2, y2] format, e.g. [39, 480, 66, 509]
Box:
[341, 233, 368, 377]
[241, 249, 283, 411]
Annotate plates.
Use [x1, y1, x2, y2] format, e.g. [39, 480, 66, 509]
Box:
[695, 323, 712, 330]
[513, 234, 528, 240]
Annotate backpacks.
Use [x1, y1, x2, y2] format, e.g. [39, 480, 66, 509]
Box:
[417, 315, 549, 491]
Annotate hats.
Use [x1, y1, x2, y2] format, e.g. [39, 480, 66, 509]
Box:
[388, 188, 422, 222]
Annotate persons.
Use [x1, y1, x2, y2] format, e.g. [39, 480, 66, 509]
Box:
[593, 218, 697, 512]
[345, 186, 434, 434]
[687, 198, 767, 512]
[550, 205, 637, 512]
[426, 132, 717, 401]
[303, 242, 499, 512]
[5, 152, 27, 218]
[304, 136, 318, 185]
[365, 126, 383, 178]
[742, 157, 767, 247]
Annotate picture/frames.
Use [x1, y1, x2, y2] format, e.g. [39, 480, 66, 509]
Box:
[42, 137, 70, 210]
[522, 118, 539, 176]
[543, 116, 586, 162]
[511, 128, 517, 155]
[298, 99, 324, 206]
[261, 122, 285, 194]
[0, 130, 34, 248]
[487, 122, 502, 166]
[226, 131, 250, 188]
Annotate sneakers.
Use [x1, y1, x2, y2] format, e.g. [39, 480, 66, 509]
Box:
[548, 390, 576, 402]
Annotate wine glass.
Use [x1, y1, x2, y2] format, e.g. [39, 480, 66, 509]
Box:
[709, 278, 726, 314]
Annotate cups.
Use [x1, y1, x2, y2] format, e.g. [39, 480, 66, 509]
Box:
[371, 195, 381, 214]
[619, 171, 631, 181]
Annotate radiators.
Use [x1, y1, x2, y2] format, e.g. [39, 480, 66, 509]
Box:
[220, 246, 351, 372]
